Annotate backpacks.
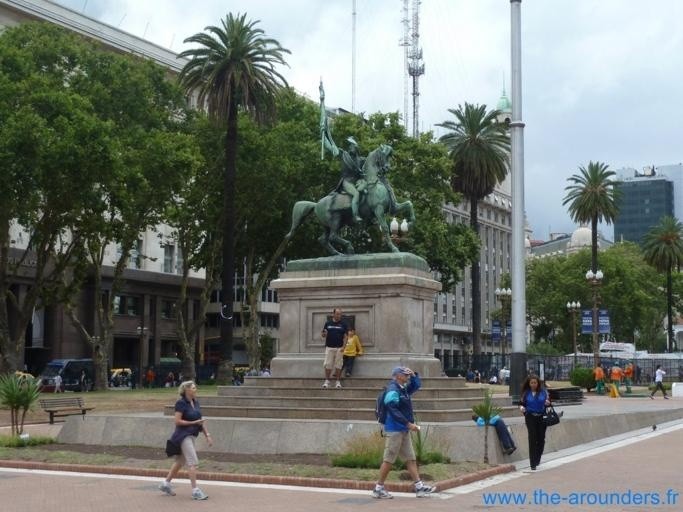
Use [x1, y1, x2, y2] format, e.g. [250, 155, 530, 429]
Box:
[375, 382, 402, 424]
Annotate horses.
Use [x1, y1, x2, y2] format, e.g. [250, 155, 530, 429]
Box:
[285, 143, 417, 255]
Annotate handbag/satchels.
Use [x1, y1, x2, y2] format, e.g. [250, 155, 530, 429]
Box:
[542, 405, 560, 426]
[165, 440, 182, 457]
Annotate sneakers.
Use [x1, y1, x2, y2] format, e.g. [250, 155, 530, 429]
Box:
[158, 482, 176, 496]
[372, 487, 393, 499]
[191, 489, 208, 500]
[322, 380, 330, 388]
[415, 482, 436, 497]
[335, 380, 341, 388]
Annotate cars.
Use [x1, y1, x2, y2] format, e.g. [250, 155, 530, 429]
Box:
[497, 369, 510, 385]
[108, 367, 132, 387]
[232, 367, 250, 381]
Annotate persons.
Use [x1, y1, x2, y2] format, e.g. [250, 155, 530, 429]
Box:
[332, 326, 364, 377]
[591, 360, 668, 400]
[158, 381, 214, 500]
[465, 365, 511, 385]
[317, 124, 366, 225]
[53, 366, 270, 395]
[471, 414, 517, 454]
[520, 374, 550, 470]
[370, 367, 437, 500]
[320, 306, 348, 387]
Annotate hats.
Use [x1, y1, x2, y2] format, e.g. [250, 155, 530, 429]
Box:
[392, 367, 409, 375]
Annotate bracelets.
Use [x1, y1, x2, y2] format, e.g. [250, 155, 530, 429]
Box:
[205, 433, 210, 437]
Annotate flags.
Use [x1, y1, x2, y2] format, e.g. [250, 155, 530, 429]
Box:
[319, 82, 339, 158]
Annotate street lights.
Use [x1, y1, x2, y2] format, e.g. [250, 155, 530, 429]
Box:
[584, 270, 604, 372]
[493, 288, 511, 371]
[565, 300, 582, 367]
[137, 326, 148, 390]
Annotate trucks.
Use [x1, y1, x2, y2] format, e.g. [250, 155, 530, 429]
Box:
[130, 358, 181, 388]
[35, 358, 111, 391]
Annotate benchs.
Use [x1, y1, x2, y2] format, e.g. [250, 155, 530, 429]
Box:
[36, 395, 95, 425]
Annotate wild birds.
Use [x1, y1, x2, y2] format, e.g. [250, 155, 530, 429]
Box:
[557, 411, 563, 416]
[652, 423, 657, 431]
